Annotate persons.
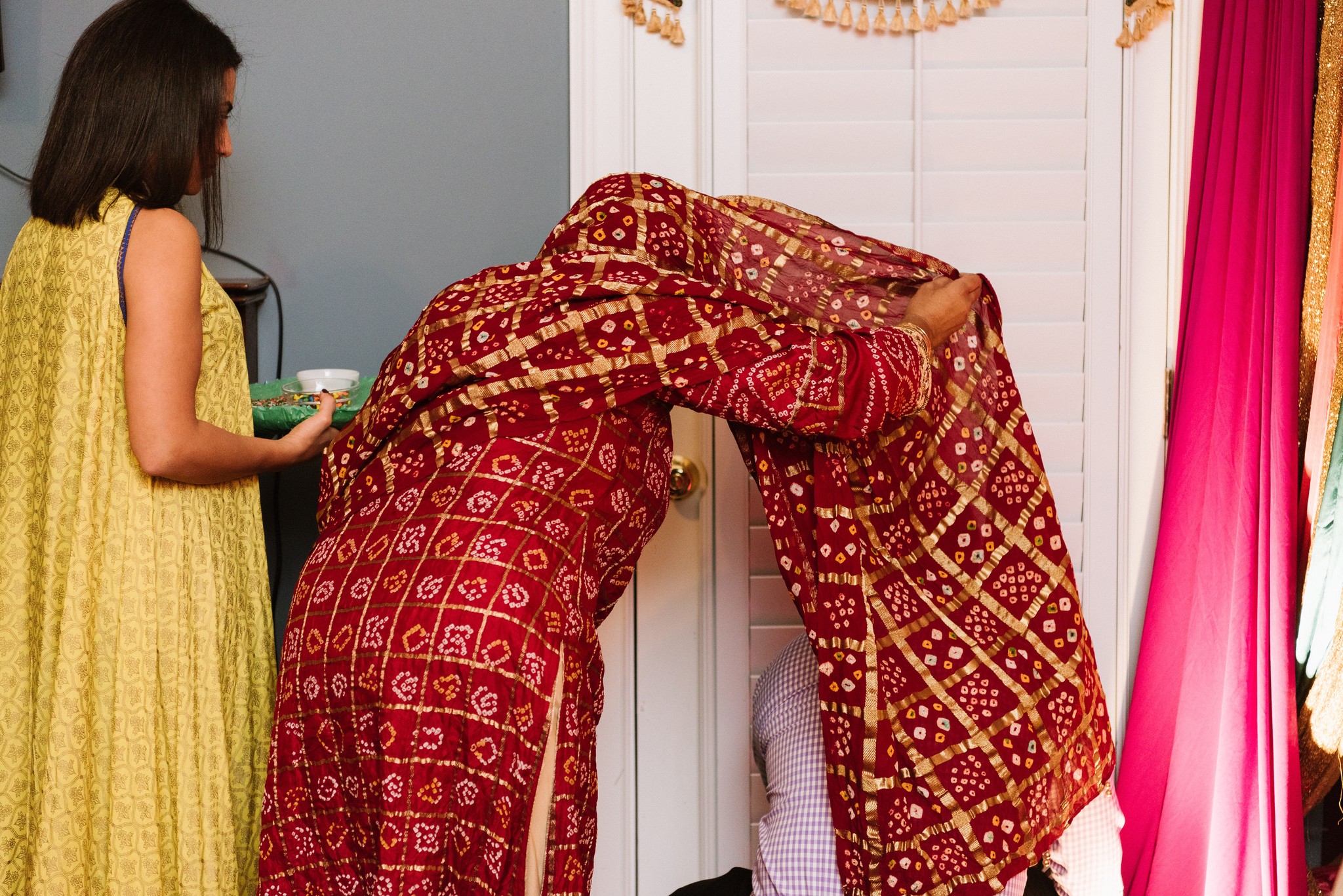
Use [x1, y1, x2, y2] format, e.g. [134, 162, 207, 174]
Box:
[0, 0, 341, 896]
[748, 630, 1128, 896]
[253, 159, 983, 895]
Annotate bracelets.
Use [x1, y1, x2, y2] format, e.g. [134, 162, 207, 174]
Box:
[891, 322, 932, 360]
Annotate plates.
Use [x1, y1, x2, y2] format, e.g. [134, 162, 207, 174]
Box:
[244, 375, 380, 431]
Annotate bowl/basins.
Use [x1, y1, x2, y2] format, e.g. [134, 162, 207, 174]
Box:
[296, 368, 360, 392]
[281, 377, 359, 411]
